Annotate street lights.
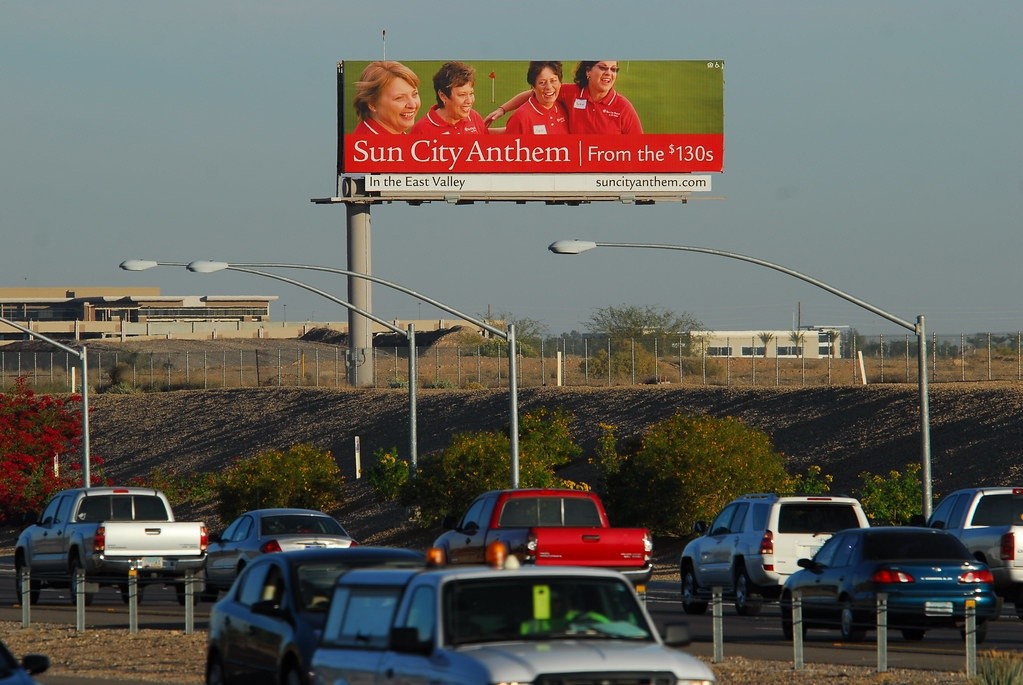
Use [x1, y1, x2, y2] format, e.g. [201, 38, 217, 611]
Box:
[548, 239, 933, 527]
[119, 258, 418, 488]
[187, 258, 523, 490]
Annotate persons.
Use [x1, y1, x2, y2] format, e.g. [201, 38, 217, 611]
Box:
[408, 62, 488, 135]
[484, 61, 642, 134]
[505, 61, 569, 134]
[353, 61, 421, 134]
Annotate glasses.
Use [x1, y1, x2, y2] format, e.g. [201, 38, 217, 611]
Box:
[596, 63, 620, 73]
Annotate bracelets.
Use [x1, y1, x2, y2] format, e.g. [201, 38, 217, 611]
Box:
[499, 107, 505, 115]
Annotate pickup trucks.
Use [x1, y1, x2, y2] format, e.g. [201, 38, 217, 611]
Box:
[431, 486, 654, 592]
[14, 485, 212, 605]
[909, 486, 1023, 624]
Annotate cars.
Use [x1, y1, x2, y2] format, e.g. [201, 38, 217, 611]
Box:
[0, 639, 50, 685]
[203, 547, 436, 685]
[198, 508, 361, 604]
[780, 525, 999, 643]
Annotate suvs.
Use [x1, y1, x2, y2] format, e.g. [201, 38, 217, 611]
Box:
[303, 564, 717, 685]
[680, 492, 871, 616]
